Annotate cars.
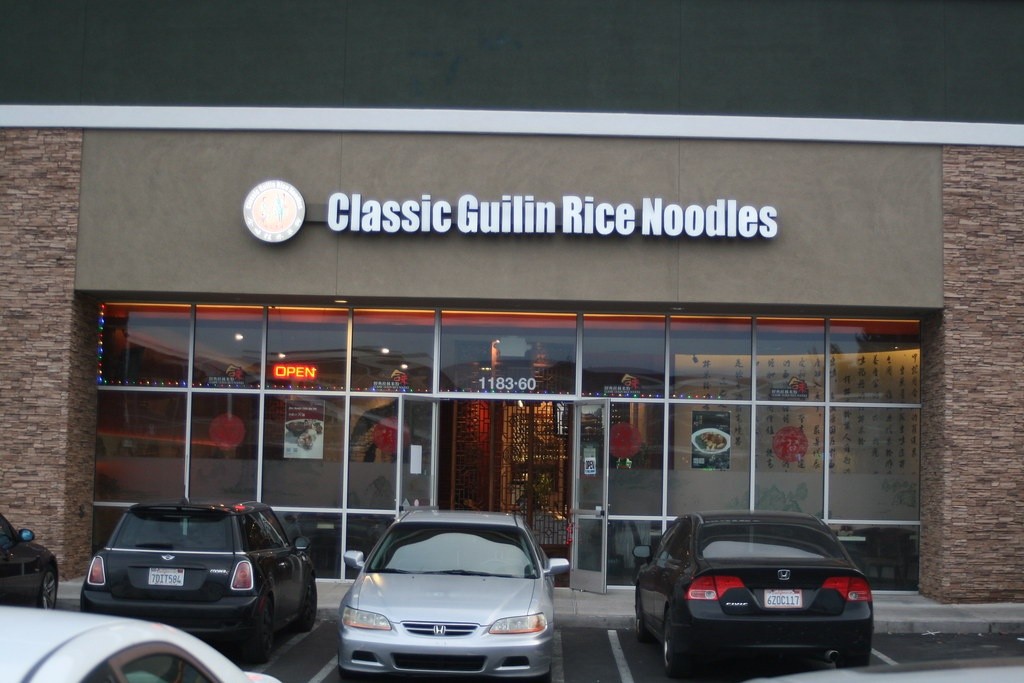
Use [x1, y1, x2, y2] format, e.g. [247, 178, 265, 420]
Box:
[632, 511, 873, 680]
[81, 499, 318, 665]
[0, 513, 59, 610]
[1, 602, 284, 682]
[337, 508, 570, 683]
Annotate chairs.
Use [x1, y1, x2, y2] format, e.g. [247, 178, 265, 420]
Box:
[861, 529, 908, 590]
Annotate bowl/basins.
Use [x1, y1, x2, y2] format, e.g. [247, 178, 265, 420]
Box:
[691, 428, 730, 454]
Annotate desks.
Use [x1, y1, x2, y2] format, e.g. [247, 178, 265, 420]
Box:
[837, 535, 867, 541]
